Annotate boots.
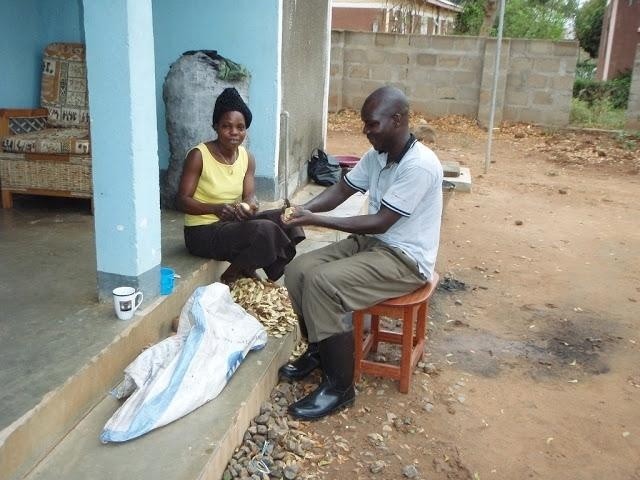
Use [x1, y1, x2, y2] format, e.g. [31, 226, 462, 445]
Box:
[279, 313, 323, 381]
[286, 330, 355, 419]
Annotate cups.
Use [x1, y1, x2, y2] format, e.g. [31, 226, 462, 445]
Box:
[112, 286, 144, 321]
[160, 267, 176, 295]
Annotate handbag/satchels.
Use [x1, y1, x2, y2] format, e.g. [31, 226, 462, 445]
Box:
[308, 148, 342, 187]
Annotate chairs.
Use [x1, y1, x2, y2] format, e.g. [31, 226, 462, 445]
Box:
[1, 41, 94, 215]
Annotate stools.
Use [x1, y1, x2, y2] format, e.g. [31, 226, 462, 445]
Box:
[349, 270, 440, 394]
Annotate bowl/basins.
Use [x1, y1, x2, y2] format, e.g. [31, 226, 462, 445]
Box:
[333, 155, 361, 168]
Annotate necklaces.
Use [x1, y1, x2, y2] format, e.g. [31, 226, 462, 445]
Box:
[215, 144, 237, 175]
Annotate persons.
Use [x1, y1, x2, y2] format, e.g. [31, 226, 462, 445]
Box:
[175, 88, 306, 283]
[275, 85, 443, 422]
